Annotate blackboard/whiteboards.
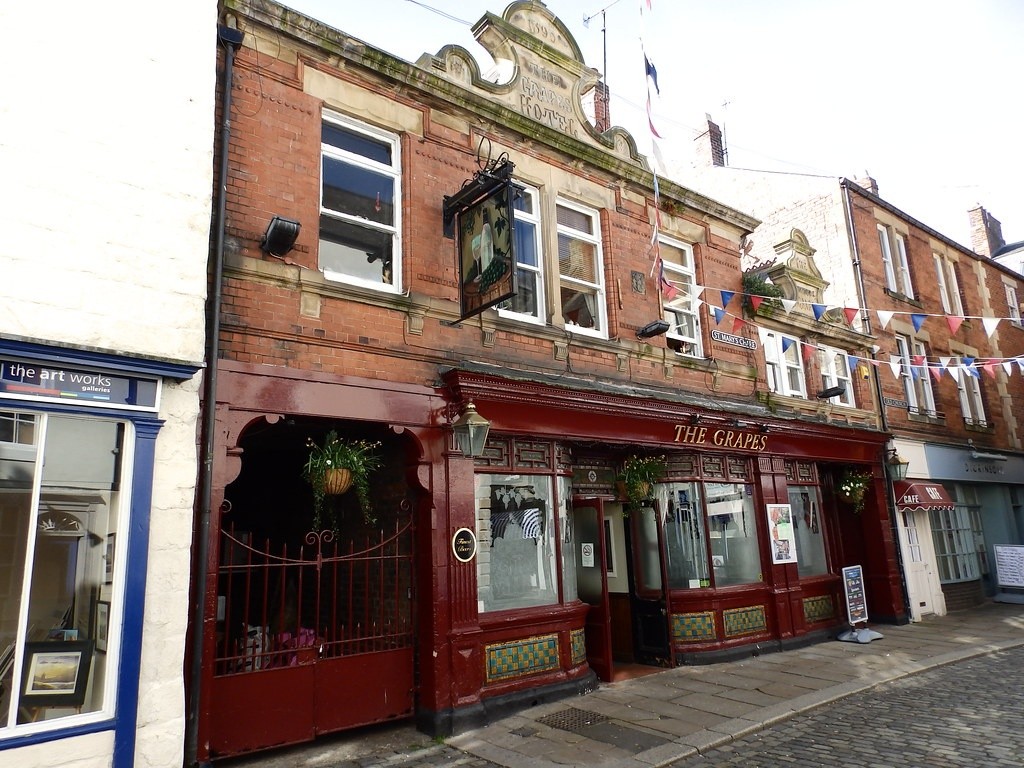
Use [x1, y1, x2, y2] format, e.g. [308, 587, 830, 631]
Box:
[993, 544, 1024, 587]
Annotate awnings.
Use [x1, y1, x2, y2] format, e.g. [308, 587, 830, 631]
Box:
[893, 479, 956, 511]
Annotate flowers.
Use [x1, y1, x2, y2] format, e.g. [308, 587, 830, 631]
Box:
[621, 455, 668, 501]
[843, 472, 873, 505]
[302, 436, 384, 478]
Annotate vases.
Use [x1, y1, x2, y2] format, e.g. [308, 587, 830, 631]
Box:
[319, 469, 352, 493]
[628, 477, 649, 502]
[849, 487, 864, 501]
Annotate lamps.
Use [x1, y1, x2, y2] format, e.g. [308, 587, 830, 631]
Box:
[261, 215, 302, 253]
[447, 399, 491, 458]
[818, 385, 845, 401]
[881, 448, 910, 482]
[635, 321, 670, 340]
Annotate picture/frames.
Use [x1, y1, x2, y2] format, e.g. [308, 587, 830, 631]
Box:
[19, 641, 95, 707]
[105, 532, 116, 585]
[93, 600, 110, 654]
